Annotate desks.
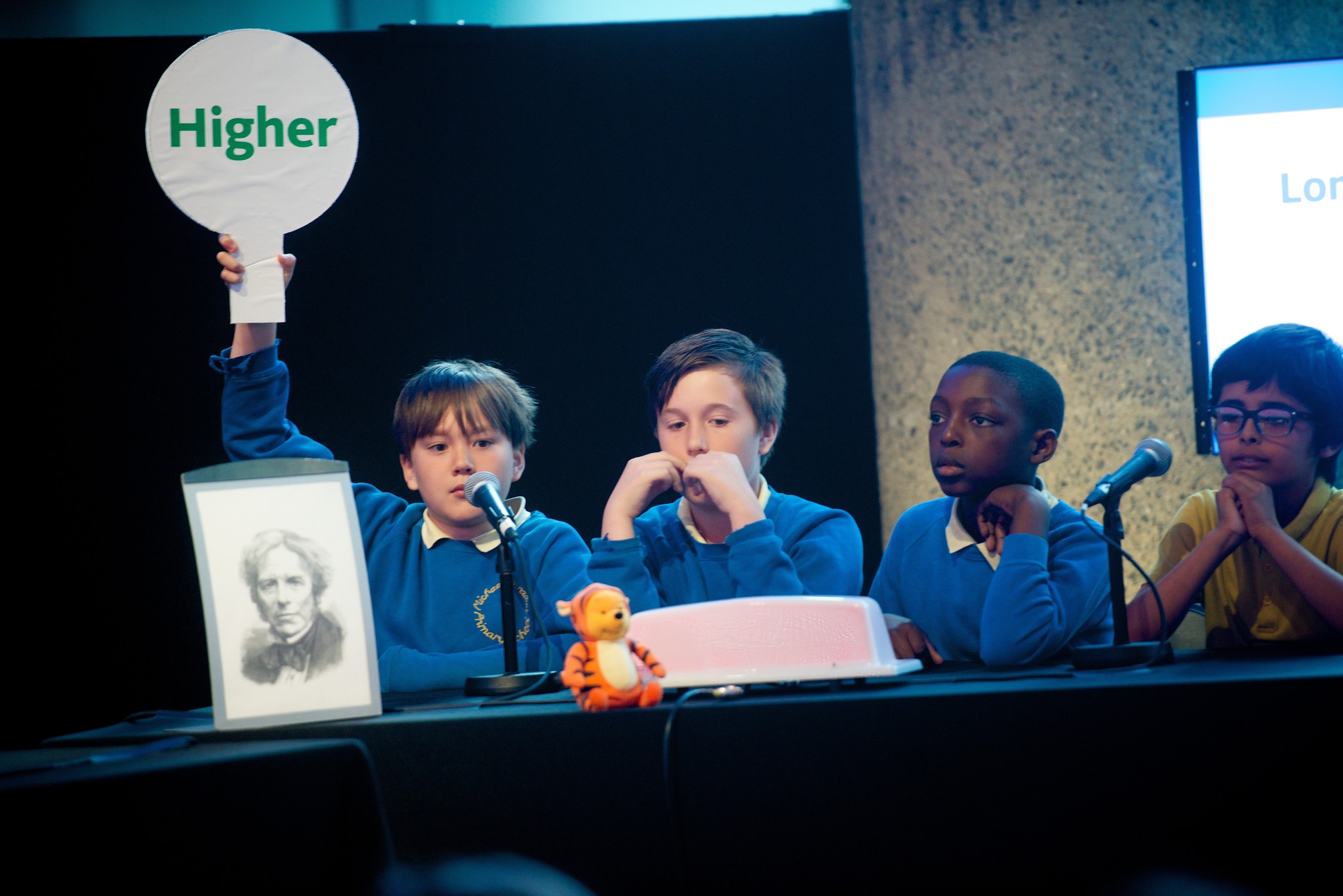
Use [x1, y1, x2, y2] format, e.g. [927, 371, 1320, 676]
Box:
[38, 654, 1343, 896]
[0, 738, 394, 896]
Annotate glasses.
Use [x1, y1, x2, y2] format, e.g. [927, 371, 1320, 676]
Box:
[1208, 404, 1316, 436]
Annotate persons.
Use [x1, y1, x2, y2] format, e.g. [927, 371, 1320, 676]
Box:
[589, 328, 864, 614]
[868, 351, 1113, 668]
[1125, 323, 1343, 646]
[211, 233, 592, 695]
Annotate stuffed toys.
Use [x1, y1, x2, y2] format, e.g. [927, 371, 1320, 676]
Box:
[556, 582, 667, 712]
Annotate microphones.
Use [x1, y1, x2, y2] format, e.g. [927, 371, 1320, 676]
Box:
[1083, 439, 1173, 508]
[465, 471, 518, 539]
